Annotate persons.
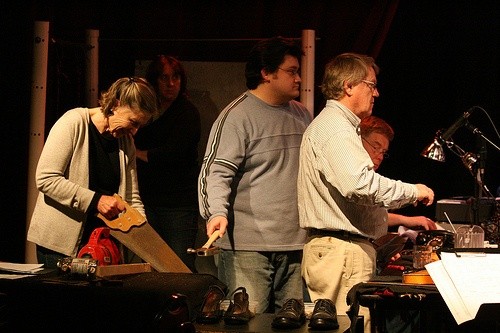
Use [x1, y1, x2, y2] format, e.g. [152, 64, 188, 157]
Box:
[298, 53, 434, 333]
[27, 78, 158, 270]
[134, 54, 201, 272]
[198, 37, 312, 313]
[359, 115, 437, 230]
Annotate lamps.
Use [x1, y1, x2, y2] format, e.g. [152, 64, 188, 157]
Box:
[421, 126, 500, 217]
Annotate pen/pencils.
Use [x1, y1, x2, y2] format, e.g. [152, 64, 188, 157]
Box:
[459, 231, 466, 242]
[444, 212, 457, 233]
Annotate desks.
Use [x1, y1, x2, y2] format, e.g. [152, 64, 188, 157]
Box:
[193, 314, 364, 333]
[346, 281, 446, 333]
[0, 271, 228, 333]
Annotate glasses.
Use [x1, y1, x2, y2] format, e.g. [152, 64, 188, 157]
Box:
[360, 135, 386, 155]
[278, 65, 298, 75]
[359, 78, 376, 92]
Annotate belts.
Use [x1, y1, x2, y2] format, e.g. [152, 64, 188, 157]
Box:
[308, 228, 376, 244]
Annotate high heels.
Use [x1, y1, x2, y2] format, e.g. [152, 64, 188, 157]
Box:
[224, 287, 250, 326]
[200, 284, 226, 325]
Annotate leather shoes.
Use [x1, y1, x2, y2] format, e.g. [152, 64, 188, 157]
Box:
[307, 298, 339, 330]
[273, 298, 306, 329]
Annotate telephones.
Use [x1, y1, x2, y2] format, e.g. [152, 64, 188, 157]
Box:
[416, 230, 454, 254]
